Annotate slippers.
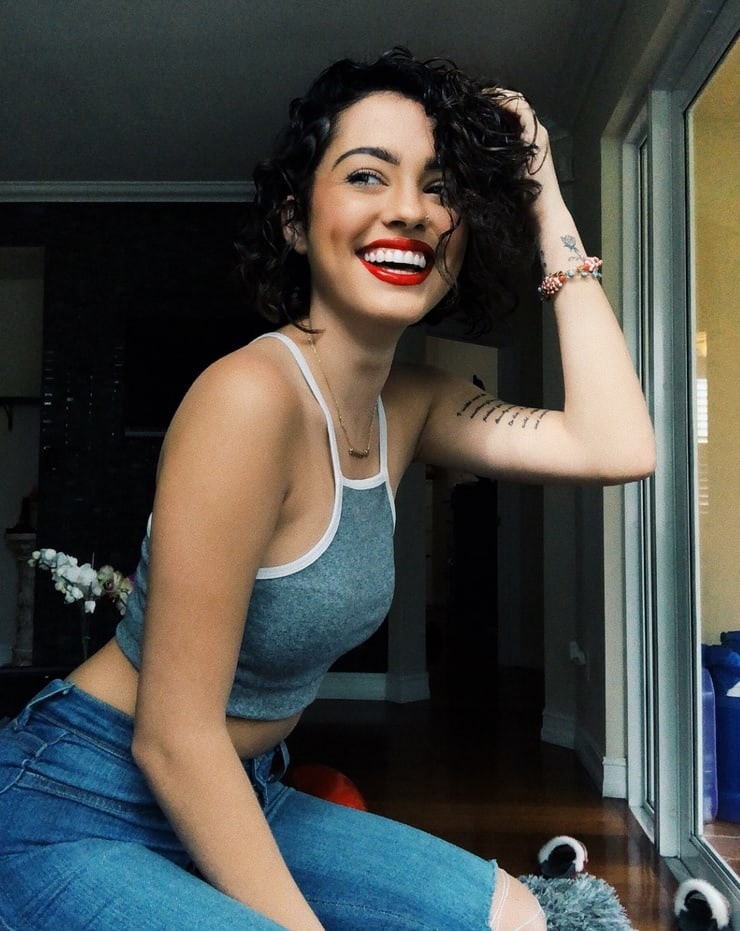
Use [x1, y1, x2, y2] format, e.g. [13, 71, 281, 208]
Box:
[672, 878, 730, 931]
[539, 835, 588, 879]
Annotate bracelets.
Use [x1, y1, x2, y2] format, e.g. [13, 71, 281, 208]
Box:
[538, 256, 604, 302]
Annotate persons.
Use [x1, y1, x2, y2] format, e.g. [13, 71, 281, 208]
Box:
[0, 47, 660, 931]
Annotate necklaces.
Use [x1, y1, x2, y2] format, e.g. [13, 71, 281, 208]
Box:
[308, 329, 378, 458]
